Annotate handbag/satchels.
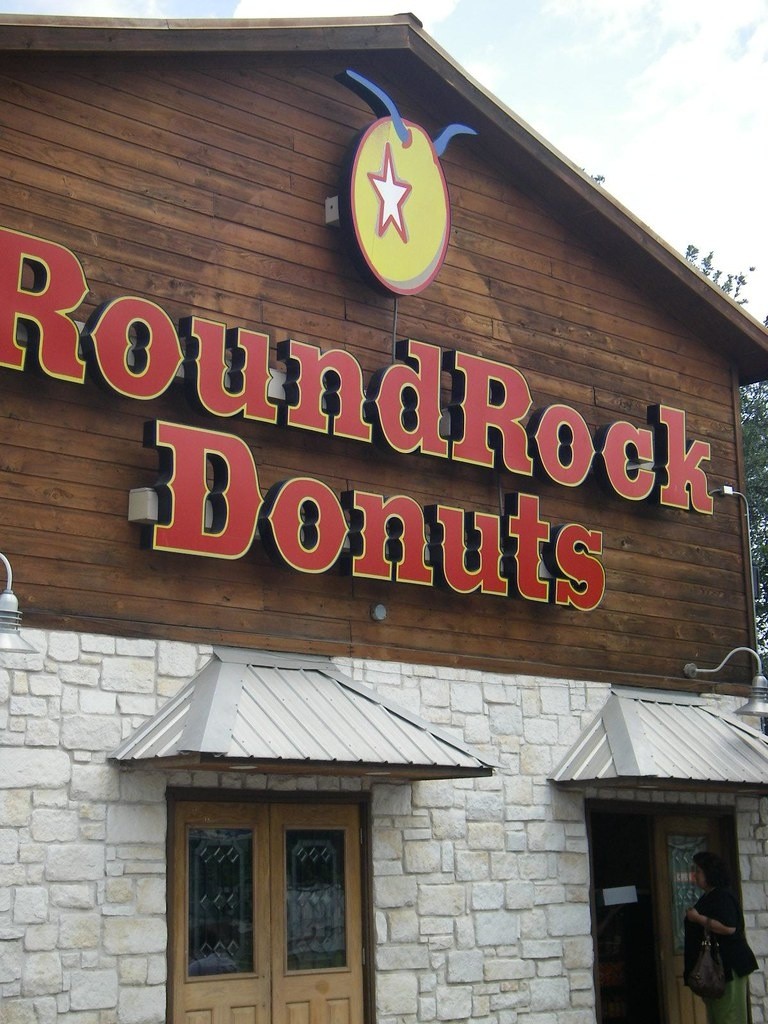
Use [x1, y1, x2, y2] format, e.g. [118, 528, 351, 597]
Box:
[687, 917, 725, 998]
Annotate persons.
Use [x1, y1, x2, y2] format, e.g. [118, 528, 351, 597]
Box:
[595, 882, 627, 1024]
[683, 852, 758, 1024]
